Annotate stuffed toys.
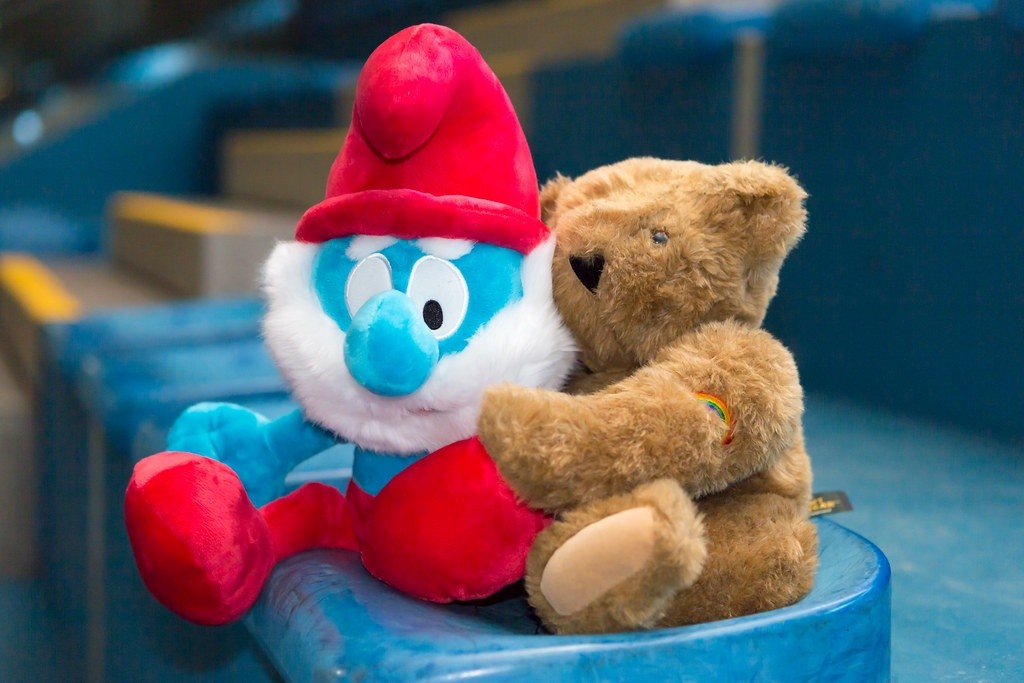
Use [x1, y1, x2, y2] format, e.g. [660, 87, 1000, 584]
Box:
[127, 25, 582, 626]
[478, 155, 820, 633]
